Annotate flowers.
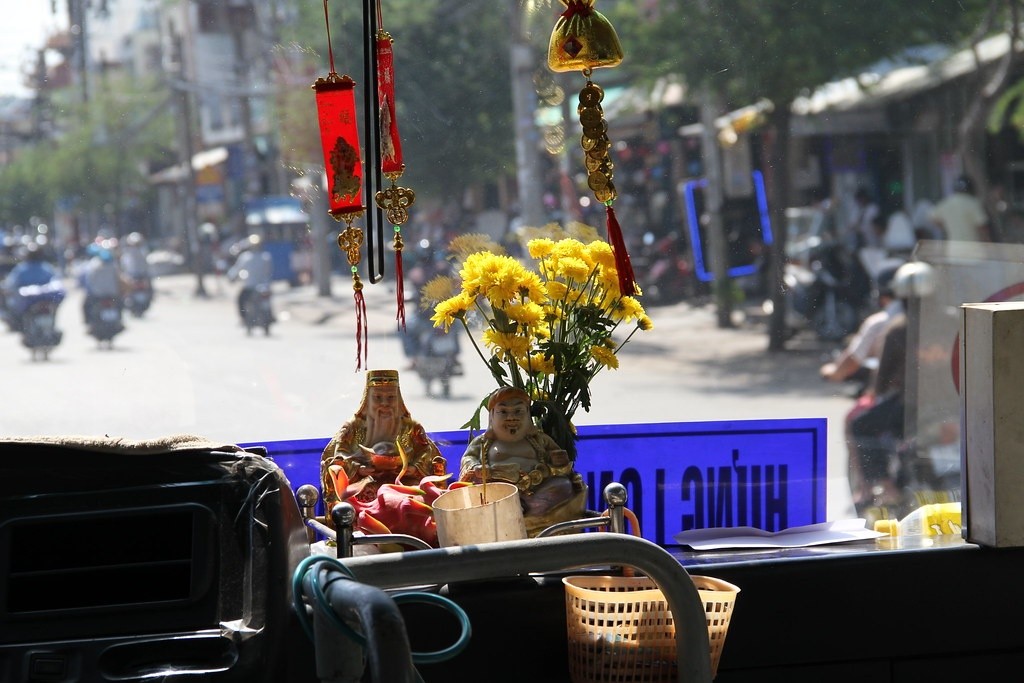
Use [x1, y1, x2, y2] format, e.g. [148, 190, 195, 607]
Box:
[419, 221, 651, 463]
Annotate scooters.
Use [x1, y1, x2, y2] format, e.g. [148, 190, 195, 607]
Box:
[0, 283, 62, 361]
[81, 282, 126, 347]
[144, 295, 147, 297]
[230, 272, 276, 337]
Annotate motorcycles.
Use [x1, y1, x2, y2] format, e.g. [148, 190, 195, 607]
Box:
[121, 266, 155, 321]
[259, 192, 963, 352]
[400, 285, 465, 404]
[825, 359, 961, 530]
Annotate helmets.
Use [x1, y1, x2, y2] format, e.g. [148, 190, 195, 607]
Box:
[892, 262, 934, 298]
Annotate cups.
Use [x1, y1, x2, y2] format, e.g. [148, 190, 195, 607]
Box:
[431, 481, 527, 548]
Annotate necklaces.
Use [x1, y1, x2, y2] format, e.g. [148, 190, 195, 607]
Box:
[482, 437, 544, 465]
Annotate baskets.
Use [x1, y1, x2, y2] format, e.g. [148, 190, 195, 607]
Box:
[562, 507, 741, 683]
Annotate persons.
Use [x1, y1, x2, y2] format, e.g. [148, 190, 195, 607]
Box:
[459, 386, 584, 517]
[320, 370, 447, 526]
[0, 233, 154, 334]
[228, 235, 273, 318]
[801, 175, 991, 505]
[394, 241, 463, 369]
